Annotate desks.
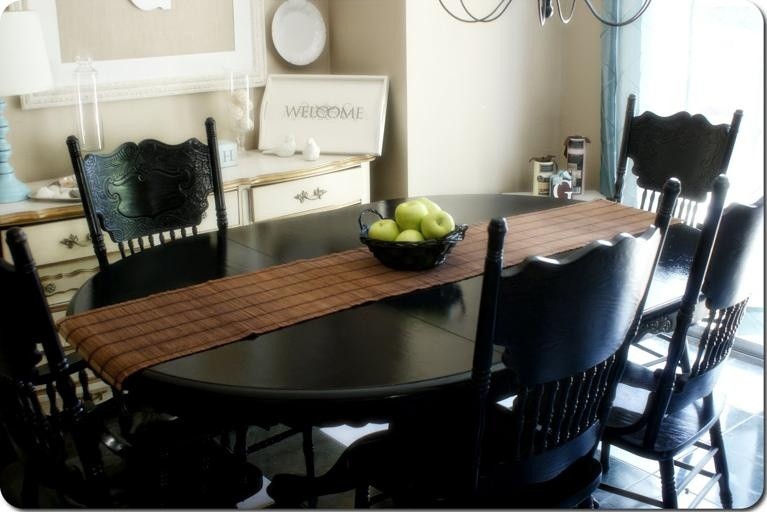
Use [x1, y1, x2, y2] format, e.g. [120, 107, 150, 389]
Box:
[57, 191, 708, 509]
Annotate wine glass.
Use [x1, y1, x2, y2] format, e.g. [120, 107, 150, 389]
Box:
[227, 70, 255, 163]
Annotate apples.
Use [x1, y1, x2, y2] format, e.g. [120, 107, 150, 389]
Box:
[368, 196, 455, 243]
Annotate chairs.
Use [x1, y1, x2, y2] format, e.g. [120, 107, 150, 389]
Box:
[596, 174, 765, 510]
[610, 95, 744, 346]
[352, 176, 683, 508]
[64, 116, 319, 508]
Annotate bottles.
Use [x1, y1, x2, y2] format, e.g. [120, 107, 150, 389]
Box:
[566, 138, 586, 195]
[72, 56, 105, 152]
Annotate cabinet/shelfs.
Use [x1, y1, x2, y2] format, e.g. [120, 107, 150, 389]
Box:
[0, 148, 378, 415]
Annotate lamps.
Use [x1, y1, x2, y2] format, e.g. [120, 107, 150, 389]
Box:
[0, 11, 54, 204]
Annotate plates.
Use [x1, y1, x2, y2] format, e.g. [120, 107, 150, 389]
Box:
[271, 0, 327, 67]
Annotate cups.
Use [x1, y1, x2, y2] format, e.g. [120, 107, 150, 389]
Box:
[533, 160, 556, 198]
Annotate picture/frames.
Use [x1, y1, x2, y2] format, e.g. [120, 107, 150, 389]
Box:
[7, 1, 268, 111]
